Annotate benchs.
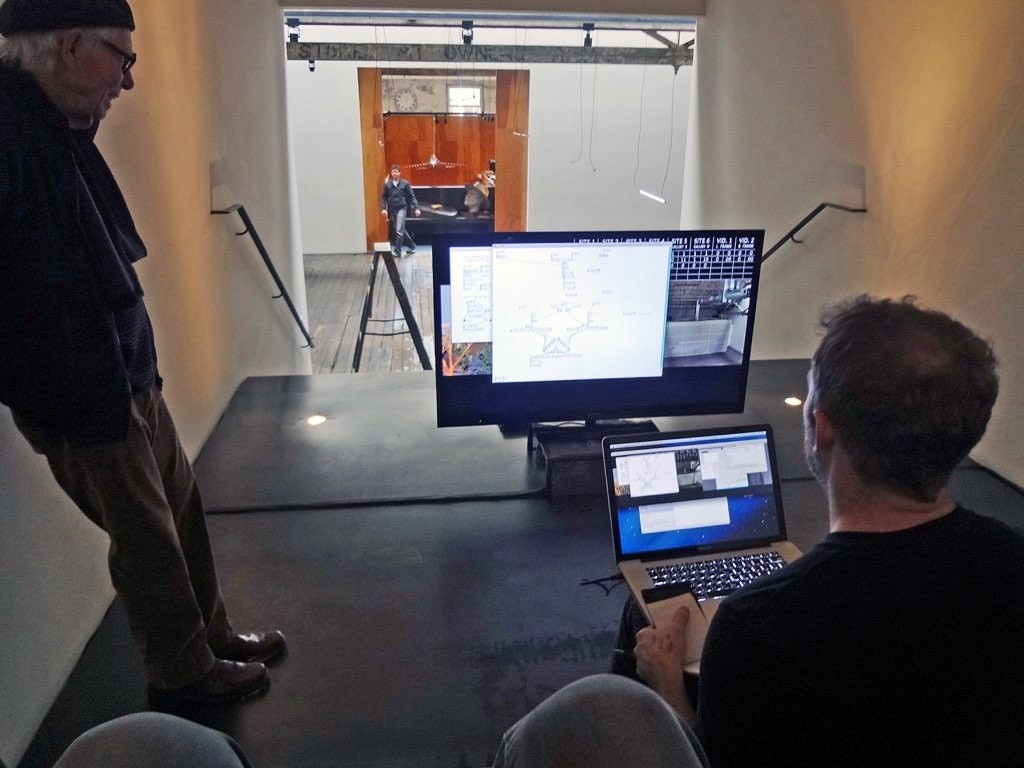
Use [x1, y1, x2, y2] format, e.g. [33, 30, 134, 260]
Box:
[389, 185, 495, 246]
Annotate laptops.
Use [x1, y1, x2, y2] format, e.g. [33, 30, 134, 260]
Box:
[602, 424, 803, 668]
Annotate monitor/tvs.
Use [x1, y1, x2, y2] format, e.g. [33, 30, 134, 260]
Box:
[430, 229, 766, 444]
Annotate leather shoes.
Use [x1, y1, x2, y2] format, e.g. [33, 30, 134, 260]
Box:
[147, 659, 271, 705]
[214, 631, 287, 663]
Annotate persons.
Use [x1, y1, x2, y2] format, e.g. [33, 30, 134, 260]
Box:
[0, 0, 290, 714]
[48, 673, 711, 768]
[609, 291, 1024, 768]
[380, 164, 422, 258]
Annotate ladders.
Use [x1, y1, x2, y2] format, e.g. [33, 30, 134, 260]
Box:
[351, 242, 433, 373]
